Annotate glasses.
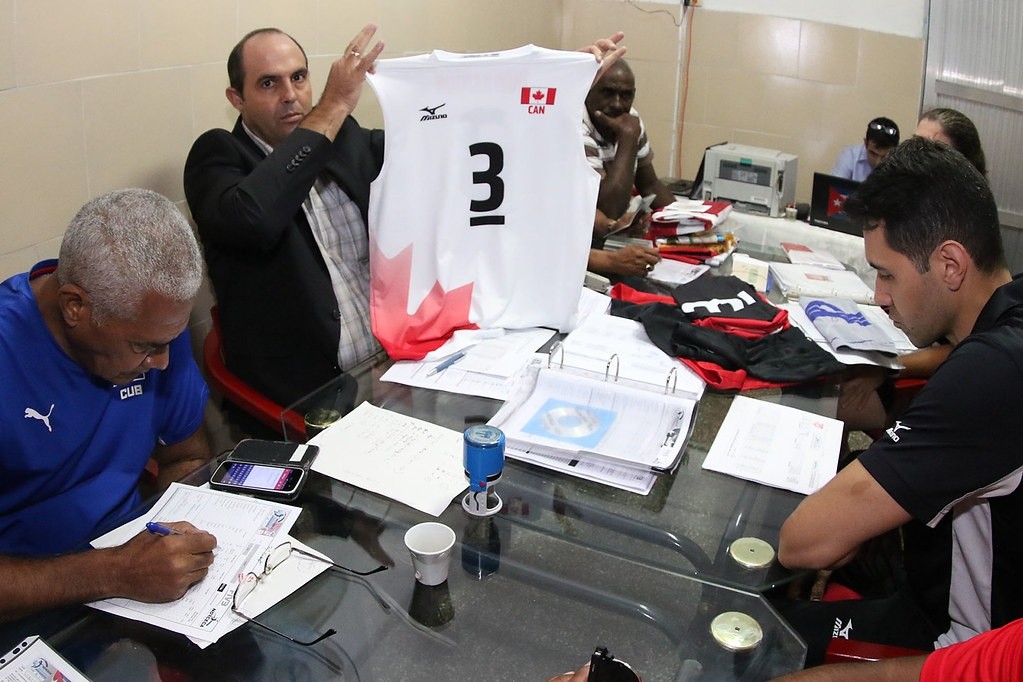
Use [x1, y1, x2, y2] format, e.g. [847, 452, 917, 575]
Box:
[231, 541, 391, 647]
[870, 122, 897, 136]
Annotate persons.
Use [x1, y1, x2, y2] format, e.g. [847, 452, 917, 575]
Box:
[546, 618, 1023, 682]
[779, 107, 1023, 668]
[0, 187, 217, 659]
[291, 489, 396, 567]
[183, 22, 678, 444]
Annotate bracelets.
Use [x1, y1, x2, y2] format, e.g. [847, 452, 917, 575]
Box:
[608, 221, 617, 232]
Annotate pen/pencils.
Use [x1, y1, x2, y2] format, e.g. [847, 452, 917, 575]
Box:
[146, 521, 222, 551]
[426, 350, 467, 377]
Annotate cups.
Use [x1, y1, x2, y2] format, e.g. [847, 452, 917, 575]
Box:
[786, 208, 798, 221]
[405, 522, 456, 586]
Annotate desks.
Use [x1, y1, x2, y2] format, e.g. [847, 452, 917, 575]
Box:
[0, 212, 878, 682]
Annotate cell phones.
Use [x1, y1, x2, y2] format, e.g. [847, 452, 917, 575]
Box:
[208, 460, 305, 497]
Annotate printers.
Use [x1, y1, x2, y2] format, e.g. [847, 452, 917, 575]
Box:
[702, 143, 798, 219]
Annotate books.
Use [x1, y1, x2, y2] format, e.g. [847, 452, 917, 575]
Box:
[483, 310, 709, 497]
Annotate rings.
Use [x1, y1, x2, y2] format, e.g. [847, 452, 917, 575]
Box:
[645, 264, 651, 270]
[350, 50, 361, 58]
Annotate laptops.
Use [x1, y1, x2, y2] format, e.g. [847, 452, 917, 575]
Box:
[809, 172, 869, 237]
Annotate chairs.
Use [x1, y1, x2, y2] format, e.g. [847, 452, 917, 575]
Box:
[197, 327, 308, 440]
